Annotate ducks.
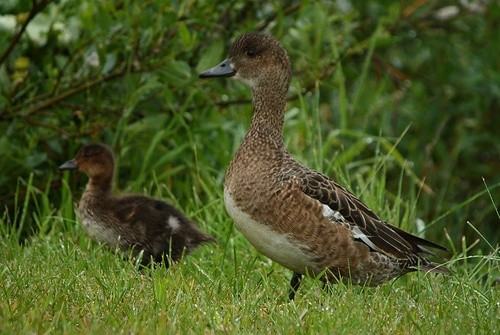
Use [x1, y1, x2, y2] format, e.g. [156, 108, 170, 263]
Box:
[58, 142, 222, 275]
[197, 31, 454, 302]
